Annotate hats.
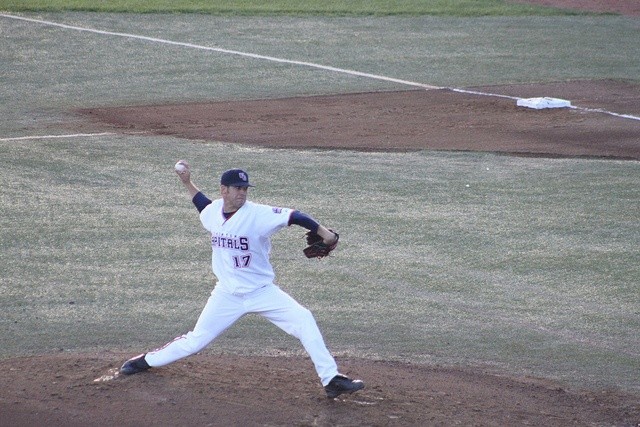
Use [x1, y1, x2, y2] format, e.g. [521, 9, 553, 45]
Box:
[221, 169, 255, 187]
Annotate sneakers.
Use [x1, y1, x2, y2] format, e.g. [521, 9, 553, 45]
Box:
[120, 352, 152, 374]
[324, 373, 365, 397]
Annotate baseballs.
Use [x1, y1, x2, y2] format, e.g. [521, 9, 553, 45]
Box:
[175, 163, 185, 172]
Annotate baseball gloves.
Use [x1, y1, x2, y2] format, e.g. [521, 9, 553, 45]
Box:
[303, 229, 339, 258]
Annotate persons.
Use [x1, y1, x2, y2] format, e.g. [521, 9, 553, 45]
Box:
[118, 158, 364, 399]
